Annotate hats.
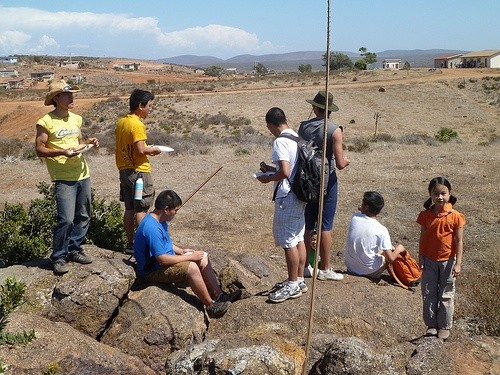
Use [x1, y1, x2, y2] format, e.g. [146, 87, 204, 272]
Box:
[306, 91, 339, 112]
[44, 79, 81, 107]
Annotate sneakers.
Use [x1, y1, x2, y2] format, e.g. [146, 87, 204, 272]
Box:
[277, 280, 308, 293]
[304, 265, 320, 277]
[268, 282, 302, 303]
[216, 290, 243, 303]
[316, 268, 345, 281]
[206, 302, 229, 318]
[53, 260, 69, 275]
[67, 253, 93, 263]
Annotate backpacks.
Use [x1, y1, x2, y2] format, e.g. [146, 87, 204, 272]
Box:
[383, 250, 422, 294]
[275, 132, 329, 203]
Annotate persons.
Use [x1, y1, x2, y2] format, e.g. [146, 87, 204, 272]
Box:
[116, 88, 161, 250]
[345, 191, 405, 281]
[36, 79, 100, 273]
[416, 177, 465, 339]
[134, 191, 242, 318]
[298, 90, 350, 280]
[257, 107, 329, 302]
[308, 231, 321, 269]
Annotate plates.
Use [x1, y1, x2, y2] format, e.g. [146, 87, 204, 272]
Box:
[253, 171, 275, 178]
[184, 251, 207, 258]
[71, 144, 94, 154]
[151, 146, 175, 152]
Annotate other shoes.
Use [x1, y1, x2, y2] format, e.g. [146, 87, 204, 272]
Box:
[126, 242, 135, 254]
[427, 328, 450, 339]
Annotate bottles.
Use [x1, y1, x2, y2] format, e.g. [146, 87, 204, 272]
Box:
[134, 173, 144, 200]
